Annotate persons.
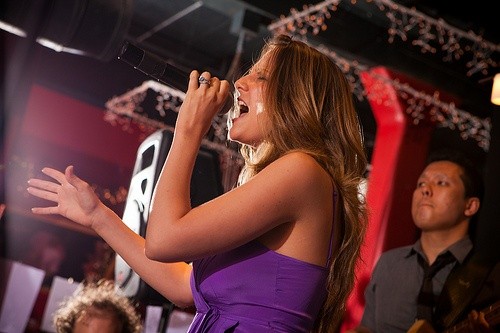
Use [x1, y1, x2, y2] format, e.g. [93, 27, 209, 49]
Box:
[26, 34, 366, 332]
[357, 150, 500, 333]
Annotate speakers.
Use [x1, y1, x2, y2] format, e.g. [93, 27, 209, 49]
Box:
[113, 128, 219, 306]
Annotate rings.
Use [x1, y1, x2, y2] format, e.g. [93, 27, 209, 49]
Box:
[199, 77, 211, 84]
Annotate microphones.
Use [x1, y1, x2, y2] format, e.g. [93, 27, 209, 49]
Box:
[117, 39, 234, 115]
